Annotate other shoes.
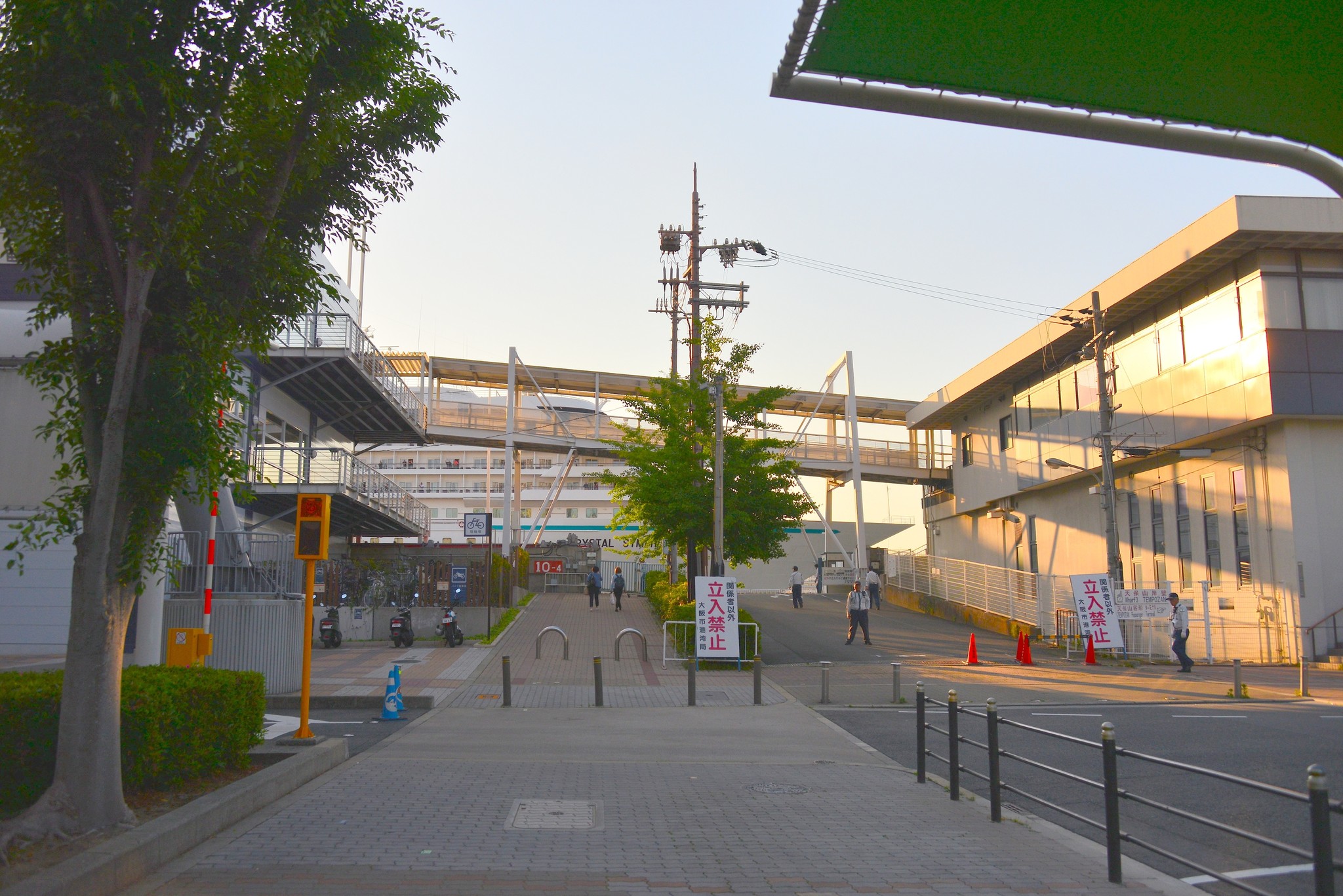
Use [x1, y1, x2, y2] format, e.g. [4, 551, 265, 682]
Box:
[619, 606, 622, 610]
[845, 642, 851, 645]
[865, 641, 872, 645]
[1177, 667, 1191, 673]
[615, 607, 618, 612]
[1185, 659, 1194, 669]
[793, 606, 798, 608]
[800, 601, 803, 608]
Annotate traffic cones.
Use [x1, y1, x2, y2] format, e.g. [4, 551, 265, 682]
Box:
[1009, 630, 1024, 662]
[371, 670, 407, 722]
[1078, 634, 1103, 666]
[1013, 633, 1037, 665]
[392, 665, 409, 712]
[961, 632, 984, 666]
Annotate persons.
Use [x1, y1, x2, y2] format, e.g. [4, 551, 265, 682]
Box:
[788, 566, 804, 609]
[403, 460, 406, 469]
[408, 460, 413, 469]
[609, 567, 626, 612]
[845, 581, 872, 645]
[379, 460, 382, 469]
[635, 558, 649, 592]
[583, 482, 588, 489]
[445, 460, 452, 469]
[419, 482, 424, 490]
[865, 565, 882, 610]
[814, 564, 819, 593]
[1166, 593, 1194, 673]
[454, 459, 458, 469]
[587, 566, 603, 611]
[375, 483, 378, 490]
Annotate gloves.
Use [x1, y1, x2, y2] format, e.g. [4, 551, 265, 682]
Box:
[1181, 631, 1186, 639]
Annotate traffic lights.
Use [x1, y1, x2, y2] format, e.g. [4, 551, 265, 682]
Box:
[294, 492, 331, 559]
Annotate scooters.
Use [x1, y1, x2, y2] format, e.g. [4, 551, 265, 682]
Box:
[435, 588, 464, 648]
[389, 592, 420, 647]
[319, 594, 348, 649]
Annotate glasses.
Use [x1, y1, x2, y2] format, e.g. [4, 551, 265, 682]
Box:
[1168, 599, 1173, 601]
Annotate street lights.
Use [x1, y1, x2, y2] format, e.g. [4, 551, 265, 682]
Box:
[1043, 456, 1124, 658]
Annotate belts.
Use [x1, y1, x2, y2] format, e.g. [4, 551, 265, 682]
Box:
[869, 582, 877, 585]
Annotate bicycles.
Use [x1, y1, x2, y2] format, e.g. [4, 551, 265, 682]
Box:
[361, 569, 484, 607]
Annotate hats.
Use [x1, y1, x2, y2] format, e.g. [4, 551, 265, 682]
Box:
[1166, 593, 1178, 600]
[854, 581, 861, 585]
[792, 566, 798, 570]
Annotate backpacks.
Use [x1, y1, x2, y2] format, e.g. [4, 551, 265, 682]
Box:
[614, 574, 624, 589]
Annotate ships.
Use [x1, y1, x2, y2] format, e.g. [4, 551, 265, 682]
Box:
[354, 378, 917, 595]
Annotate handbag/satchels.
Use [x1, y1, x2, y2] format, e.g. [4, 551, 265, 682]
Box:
[610, 592, 615, 605]
[1171, 629, 1177, 639]
[587, 576, 596, 588]
[583, 586, 589, 595]
